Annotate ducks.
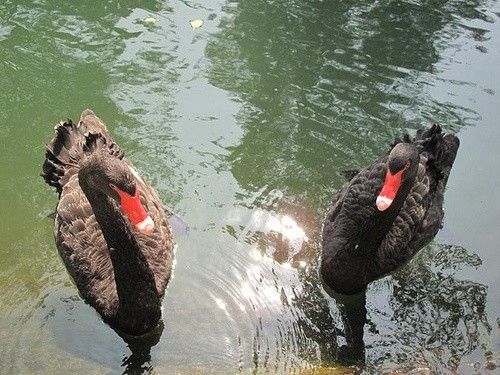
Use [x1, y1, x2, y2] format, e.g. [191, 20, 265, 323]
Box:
[39, 109, 175, 334]
[320, 123, 461, 294]
